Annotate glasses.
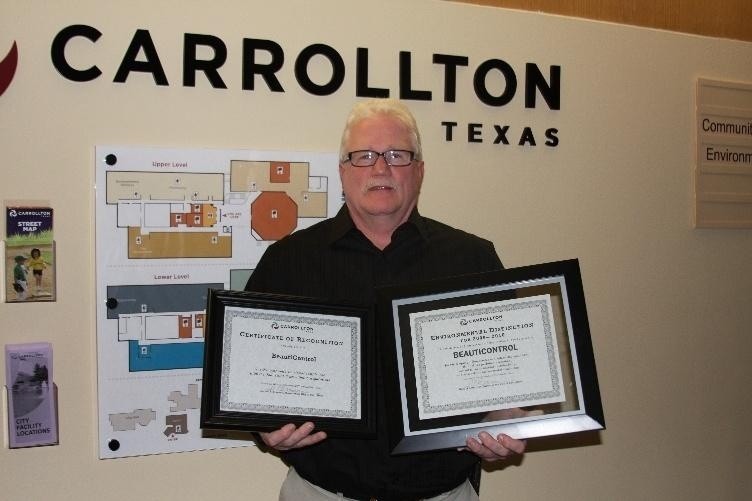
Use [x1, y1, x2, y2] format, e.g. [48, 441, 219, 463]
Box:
[343, 149, 421, 167]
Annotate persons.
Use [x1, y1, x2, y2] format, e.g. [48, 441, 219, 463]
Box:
[242, 99, 545, 501]
[11, 255, 28, 298]
[26, 247, 48, 290]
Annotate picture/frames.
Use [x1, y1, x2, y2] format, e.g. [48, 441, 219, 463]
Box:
[375, 257, 606, 455]
[200, 288, 375, 442]
